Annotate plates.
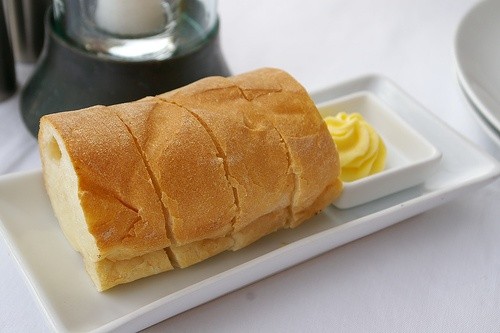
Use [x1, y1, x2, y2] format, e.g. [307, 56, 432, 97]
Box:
[1, 74, 500, 333]
[455, 0, 499, 134]
[315, 92, 442, 211]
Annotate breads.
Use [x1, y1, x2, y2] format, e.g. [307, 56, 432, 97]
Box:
[36, 70, 343, 292]
